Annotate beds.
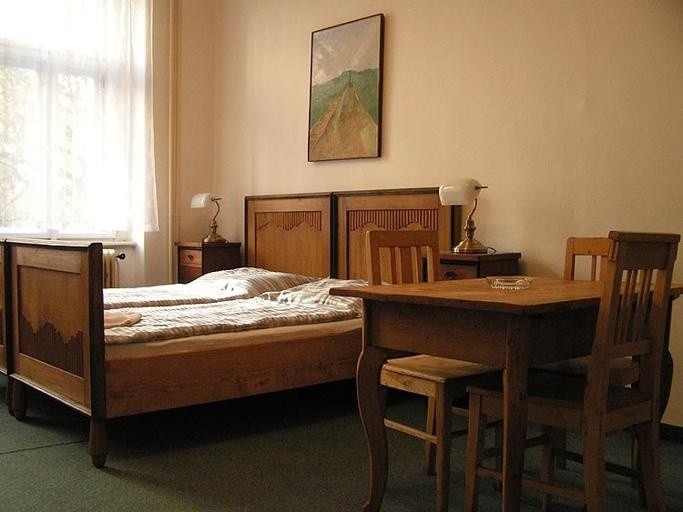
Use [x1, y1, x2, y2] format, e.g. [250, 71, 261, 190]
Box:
[7, 190, 462, 469]
[0, 193, 334, 415]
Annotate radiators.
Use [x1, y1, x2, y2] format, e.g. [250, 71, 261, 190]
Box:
[101, 249, 126, 289]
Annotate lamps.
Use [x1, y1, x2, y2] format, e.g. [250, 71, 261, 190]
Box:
[439, 179, 490, 253]
[190, 192, 226, 241]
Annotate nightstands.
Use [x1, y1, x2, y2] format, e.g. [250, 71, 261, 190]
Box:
[422, 251, 522, 282]
[174, 239, 242, 283]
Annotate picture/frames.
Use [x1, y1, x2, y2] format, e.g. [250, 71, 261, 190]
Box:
[308, 13, 384, 162]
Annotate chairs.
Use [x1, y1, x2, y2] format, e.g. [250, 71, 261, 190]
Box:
[365, 229, 501, 511]
[463, 232, 679, 512]
[493, 237, 642, 492]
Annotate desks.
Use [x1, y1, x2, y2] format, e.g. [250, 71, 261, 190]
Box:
[329, 277, 683, 511]
